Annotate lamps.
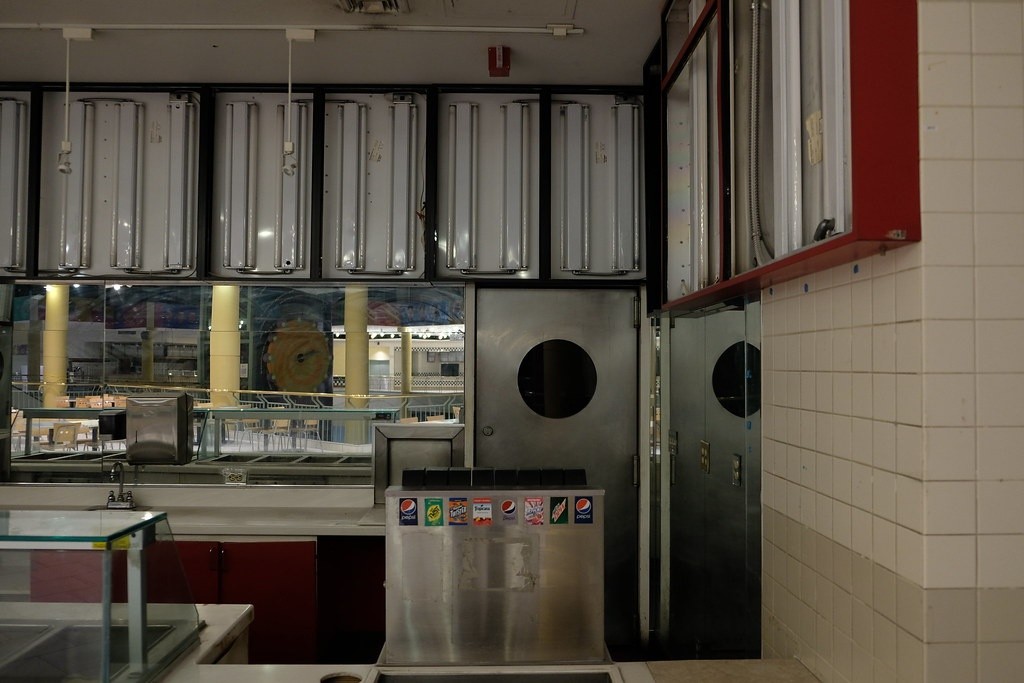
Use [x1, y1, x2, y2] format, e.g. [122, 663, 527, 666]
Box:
[55, 39, 72, 175]
[282, 38, 297, 177]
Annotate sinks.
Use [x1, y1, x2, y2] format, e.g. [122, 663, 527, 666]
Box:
[82, 505, 152, 511]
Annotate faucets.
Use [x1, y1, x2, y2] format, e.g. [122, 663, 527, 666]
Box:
[110, 462, 124, 502]
